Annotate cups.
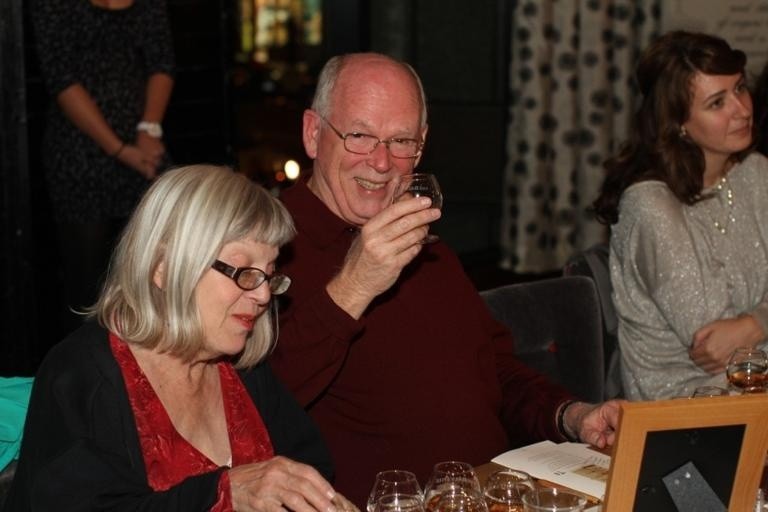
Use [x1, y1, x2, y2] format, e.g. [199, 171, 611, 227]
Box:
[727, 346, 767, 391]
[363, 462, 589, 512]
[691, 385, 729, 396]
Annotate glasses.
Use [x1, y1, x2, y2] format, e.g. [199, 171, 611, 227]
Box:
[210, 259, 292, 295]
[323, 114, 426, 159]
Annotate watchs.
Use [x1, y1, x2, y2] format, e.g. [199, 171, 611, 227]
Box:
[132, 118, 165, 142]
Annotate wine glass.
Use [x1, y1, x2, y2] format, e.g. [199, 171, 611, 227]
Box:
[393, 170, 442, 244]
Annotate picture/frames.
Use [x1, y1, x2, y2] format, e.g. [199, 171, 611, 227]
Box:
[601, 391, 768, 512]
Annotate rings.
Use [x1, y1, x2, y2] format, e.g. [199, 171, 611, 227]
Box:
[327, 489, 336, 499]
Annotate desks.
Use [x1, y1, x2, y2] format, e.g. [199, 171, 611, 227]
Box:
[365, 444, 768, 512]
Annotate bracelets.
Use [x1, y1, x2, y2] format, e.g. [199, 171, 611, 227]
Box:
[110, 138, 129, 158]
[557, 398, 585, 441]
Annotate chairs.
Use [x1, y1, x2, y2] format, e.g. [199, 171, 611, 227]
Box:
[568, 237, 621, 403]
[478, 275, 608, 405]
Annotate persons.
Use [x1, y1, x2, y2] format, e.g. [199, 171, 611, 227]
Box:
[590, 30, 768, 405]
[5, 167, 360, 511]
[23, 1, 181, 376]
[269, 48, 635, 510]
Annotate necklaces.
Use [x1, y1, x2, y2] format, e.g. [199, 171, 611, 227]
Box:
[689, 167, 735, 238]
